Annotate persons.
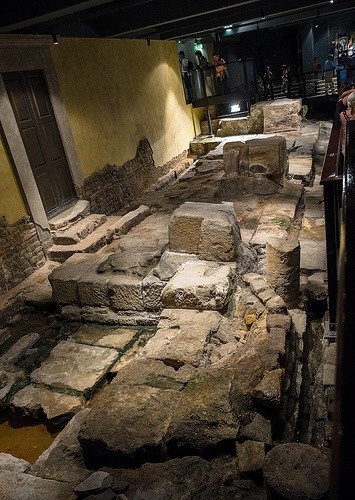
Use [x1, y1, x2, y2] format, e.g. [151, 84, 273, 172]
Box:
[337, 88, 355, 156]
[178, 50, 192, 103]
[195, 51, 214, 97]
[281, 65, 288, 93]
[210, 52, 228, 95]
[325, 54, 335, 95]
[328, 39, 355, 81]
[256, 66, 276, 101]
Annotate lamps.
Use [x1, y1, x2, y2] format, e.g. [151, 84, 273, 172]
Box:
[52, 34, 59, 45]
